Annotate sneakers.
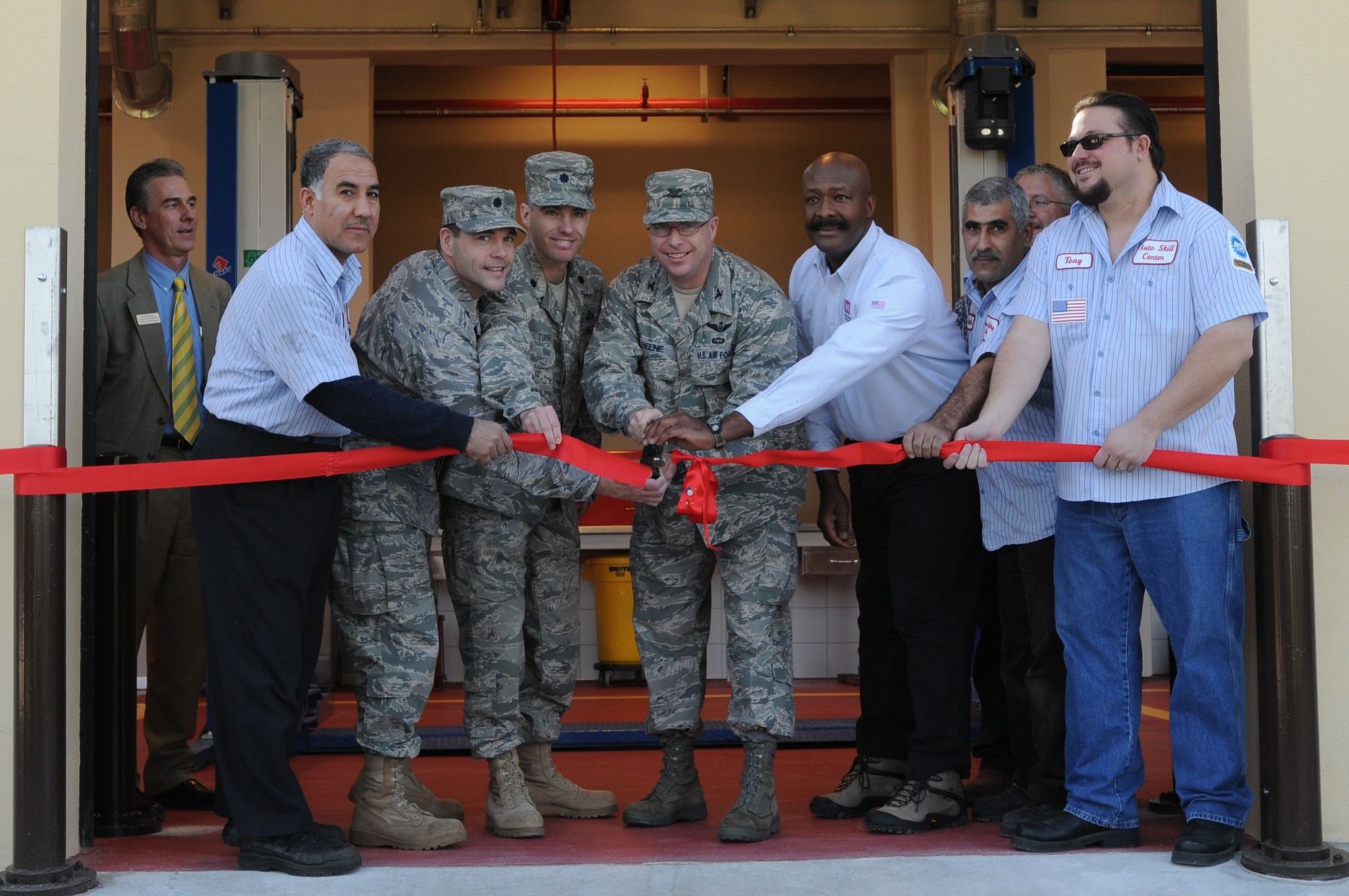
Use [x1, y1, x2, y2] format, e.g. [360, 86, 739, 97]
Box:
[863, 770, 968, 834]
[809, 754, 910, 820]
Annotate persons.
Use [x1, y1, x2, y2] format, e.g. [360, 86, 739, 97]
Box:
[582, 166, 801, 842]
[193, 135, 514, 876]
[1014, 162, 1078, 247]
[331, 186, 671, 855]
[642, 150, 970, 833]
[901, 90, 1268, 867]
[442, 149, 615, 837]
[96, 155, 232, 835]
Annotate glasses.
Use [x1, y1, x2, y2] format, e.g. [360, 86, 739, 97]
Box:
[1060, 133, 1154, 157]
[647, 215, 716, 237]
[1028, 198, 1073, 213]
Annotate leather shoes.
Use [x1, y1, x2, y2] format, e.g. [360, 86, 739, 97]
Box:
[1012, 808, 1140, 852]
[999, 795, 1068, 838]
[1171, 818, 1246, 866]
[236, 830, 363, 876]
[221, 812, 347, 847]
[971, 778, 1030, 824]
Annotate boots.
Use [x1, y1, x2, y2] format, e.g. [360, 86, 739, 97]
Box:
[718, 741, 780, 842]
[346, 745, 465, 821]
[622, 734, 708, 826]
[348, 754, 467, 850]
[516, 740, 618, 818]
[485, 748, 545, 838]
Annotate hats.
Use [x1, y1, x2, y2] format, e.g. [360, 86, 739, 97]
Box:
[439, 185, 528, 234]
[524, 151, 597, 211]
[643, 169, 714, 224]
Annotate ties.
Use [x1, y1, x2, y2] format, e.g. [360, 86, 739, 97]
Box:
[168, 276, 202, 445]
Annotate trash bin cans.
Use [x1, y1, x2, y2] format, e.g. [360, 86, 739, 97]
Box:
[582, 556, 646, 687]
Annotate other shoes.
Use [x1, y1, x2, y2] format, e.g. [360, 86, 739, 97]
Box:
[300, 696, 320, 728]
[150, 779, 215, 811]
[134, 787, 166, 818]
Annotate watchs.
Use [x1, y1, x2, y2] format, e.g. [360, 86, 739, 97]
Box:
[707, 415, 726, 450]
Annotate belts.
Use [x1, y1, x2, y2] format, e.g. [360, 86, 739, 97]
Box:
[160, 434, 192, 451]
